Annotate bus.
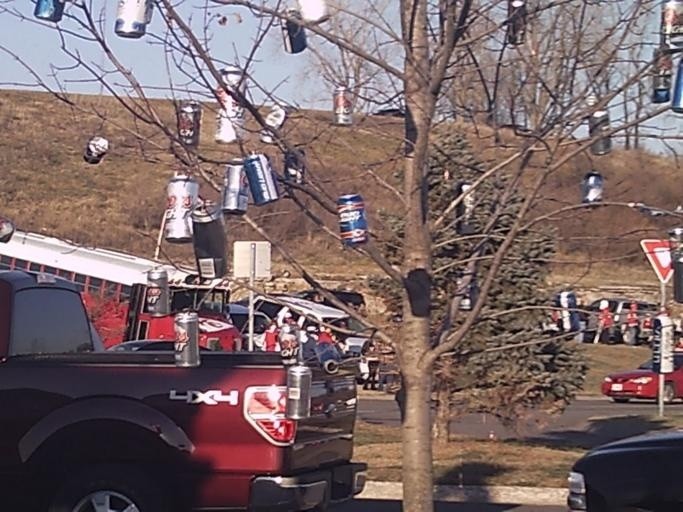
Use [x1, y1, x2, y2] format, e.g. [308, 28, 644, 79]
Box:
[0, 229, 244, 352]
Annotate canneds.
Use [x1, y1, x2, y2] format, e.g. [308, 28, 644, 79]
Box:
[32, 1, 682, 420]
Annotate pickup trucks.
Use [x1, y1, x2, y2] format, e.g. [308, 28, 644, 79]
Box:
[0, 269, 368, 512]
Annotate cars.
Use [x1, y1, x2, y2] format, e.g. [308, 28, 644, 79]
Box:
[542, 286, 682, 345]
[602, 351, 683, 404]
[567, 427, 683, 512]
[206, 290, 396, 384]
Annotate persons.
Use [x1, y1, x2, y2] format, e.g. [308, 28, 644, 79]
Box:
[576, 300, 654, 345]
[261, 312, 403, 391]
